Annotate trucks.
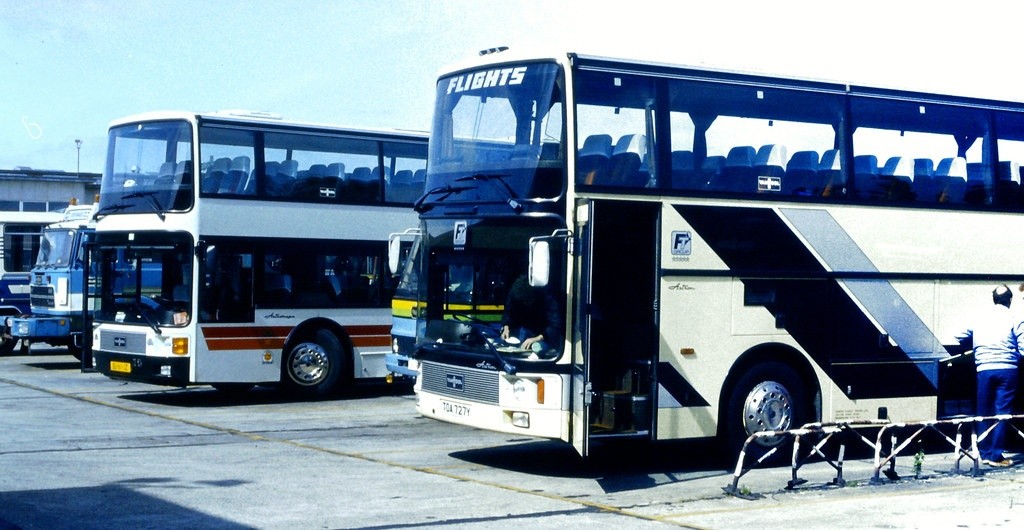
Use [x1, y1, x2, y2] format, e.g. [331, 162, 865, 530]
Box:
[11, 195, 376, 366]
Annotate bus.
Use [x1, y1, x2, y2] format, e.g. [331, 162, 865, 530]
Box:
[0, 208, 67, 356]
[415, 44, 1024, 453]
[83, 111, 551, 400]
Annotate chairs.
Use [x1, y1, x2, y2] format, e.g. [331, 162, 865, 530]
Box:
[154, 159, 194, 184]
[576, 134, 1024, 209]
[204, 156, 426, 206]
[173, 284, 190, 305]
[510, 144, 537, 161]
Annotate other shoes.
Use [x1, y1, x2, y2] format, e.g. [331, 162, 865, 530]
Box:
[983, 459, 1015, 467]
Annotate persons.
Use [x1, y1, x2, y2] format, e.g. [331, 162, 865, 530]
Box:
[952, 281, 1024, 469]
[500, 273, 561, 351]
